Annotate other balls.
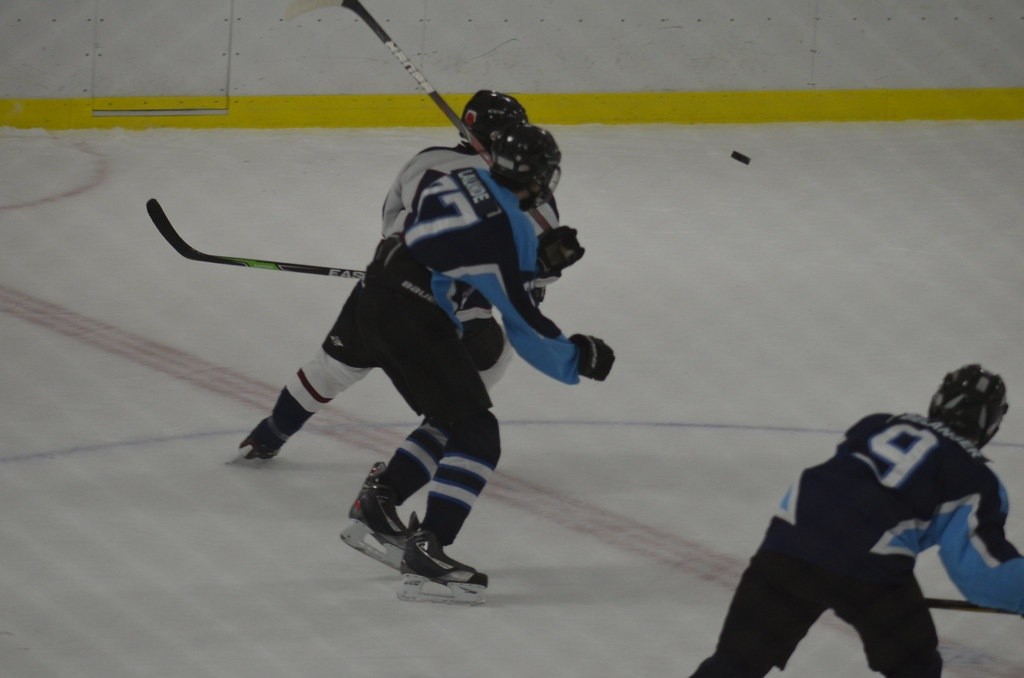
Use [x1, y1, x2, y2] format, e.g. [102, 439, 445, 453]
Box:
[731, 151, 751, 165]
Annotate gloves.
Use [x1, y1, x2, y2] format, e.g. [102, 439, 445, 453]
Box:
[569, 333, 614, 381]
[535, 224, 584, 279]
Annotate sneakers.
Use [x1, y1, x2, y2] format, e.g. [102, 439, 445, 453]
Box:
[339, 460, 409, 569]
[397, 510, 488, 605]
[222, 419, 287, 467]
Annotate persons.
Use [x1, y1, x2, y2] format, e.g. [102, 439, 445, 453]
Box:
[691, 361, 1023, 678]
[339, 126, 613, 604]
[236, 89, 558, 461]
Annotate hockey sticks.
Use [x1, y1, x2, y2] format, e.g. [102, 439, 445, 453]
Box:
[281, 0, 574, 256]
[924, 596, 1021, 616]
[145, 197, 364, 278]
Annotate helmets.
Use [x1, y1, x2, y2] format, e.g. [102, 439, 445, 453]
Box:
[489, 123, 563, 204]
[928, 363, 1008, 448]
[461, 89, 526, 143]
